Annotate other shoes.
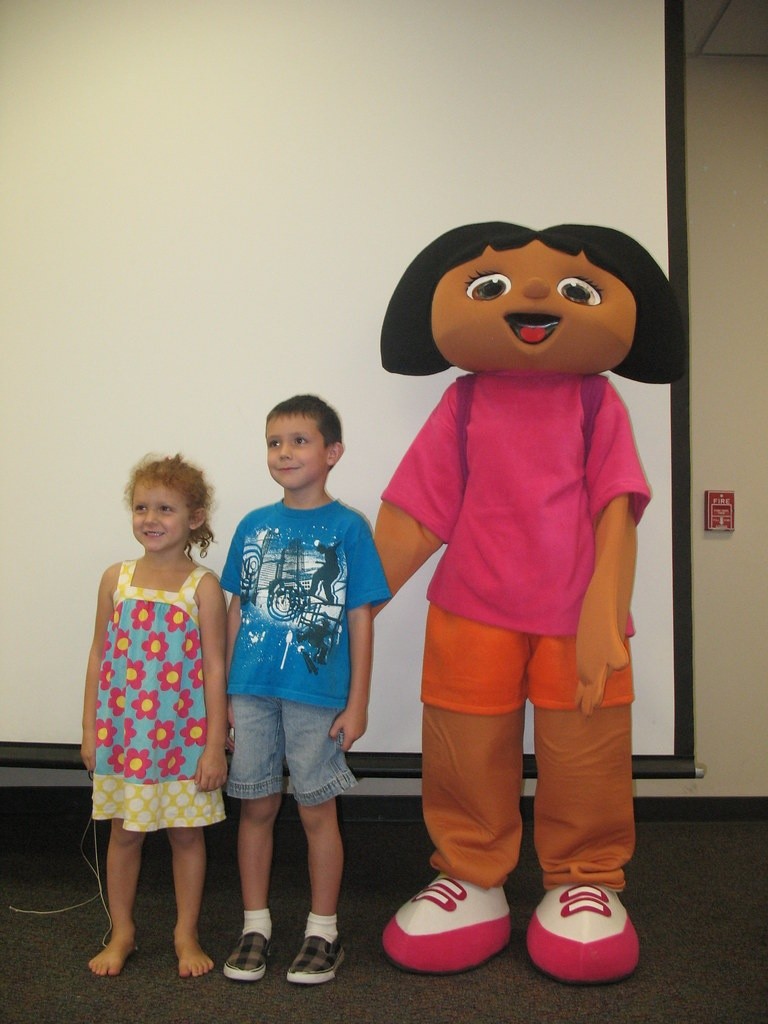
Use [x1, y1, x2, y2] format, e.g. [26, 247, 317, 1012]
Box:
[223, 930, 272, 982]
[286, 933, 345, 985]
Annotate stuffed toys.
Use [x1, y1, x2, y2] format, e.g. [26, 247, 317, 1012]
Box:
[371, 220, 689, 981]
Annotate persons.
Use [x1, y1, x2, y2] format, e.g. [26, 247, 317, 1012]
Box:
[219, 395, 393, 984]
[79, 452, 229, 976]
